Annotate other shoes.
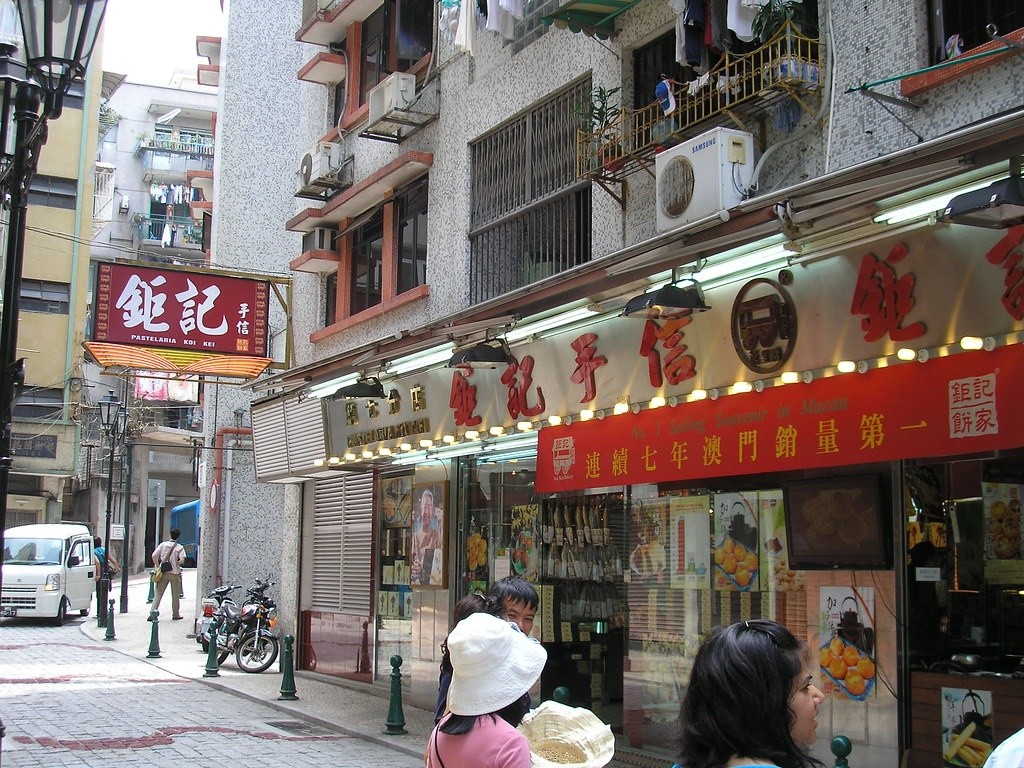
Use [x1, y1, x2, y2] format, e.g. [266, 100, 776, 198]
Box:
[172, 616, 183, 620]
[147, 615, 153, 621]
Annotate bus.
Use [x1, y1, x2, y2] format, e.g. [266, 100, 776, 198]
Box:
[170, 499, 199, 568]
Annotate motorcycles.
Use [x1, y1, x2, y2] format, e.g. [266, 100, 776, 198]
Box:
[196, 572, 278, 673]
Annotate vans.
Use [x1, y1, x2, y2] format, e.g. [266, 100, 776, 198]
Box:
[1, 523, 95, 627]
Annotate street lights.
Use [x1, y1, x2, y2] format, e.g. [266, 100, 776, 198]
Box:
[0, 1, 109, 583]
[97, 387, 123, 629]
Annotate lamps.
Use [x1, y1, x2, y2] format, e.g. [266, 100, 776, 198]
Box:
[305, 376, 362, 398]
[336, 371, 389, 401]
[445, 324, 515, 369]
[492, 302, 607, 342]
[870, 153, 1024, 230]
[384, 346, 463, 375]
[618, 240, 802, 320]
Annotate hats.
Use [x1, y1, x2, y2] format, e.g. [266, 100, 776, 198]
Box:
[447, 613, 547, 716]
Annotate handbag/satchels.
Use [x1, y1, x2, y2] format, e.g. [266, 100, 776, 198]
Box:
[152, 567, 163, 583]
[107, 566, 116, 577]
[159, 561, 172, 573]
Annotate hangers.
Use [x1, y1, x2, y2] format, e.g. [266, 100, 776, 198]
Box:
[150, 179, 191, 191]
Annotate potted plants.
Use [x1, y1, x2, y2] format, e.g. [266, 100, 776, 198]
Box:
[751, 0, 819, 92]
[572, 84, 625, 179]
[138, 131, 197, 154]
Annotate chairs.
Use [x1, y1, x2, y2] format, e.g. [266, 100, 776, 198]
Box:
[44, 548, 60, 561]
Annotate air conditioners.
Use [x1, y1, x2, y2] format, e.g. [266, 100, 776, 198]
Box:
[368, 70, 416, 136]
[655, 127, 754, 234]
[296, 141, 339, 195]
[120, 195, 128, 214]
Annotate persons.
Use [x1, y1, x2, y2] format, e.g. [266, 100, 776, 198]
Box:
[490, 575, 539, 636]
[436, 592, 532, 729]
[673, 617, 830, 768]
[147, 527, 187, 621]
[425, 611, 548, 767]
[412, 488, 443, 584]
[91, 535, 120, 619]
